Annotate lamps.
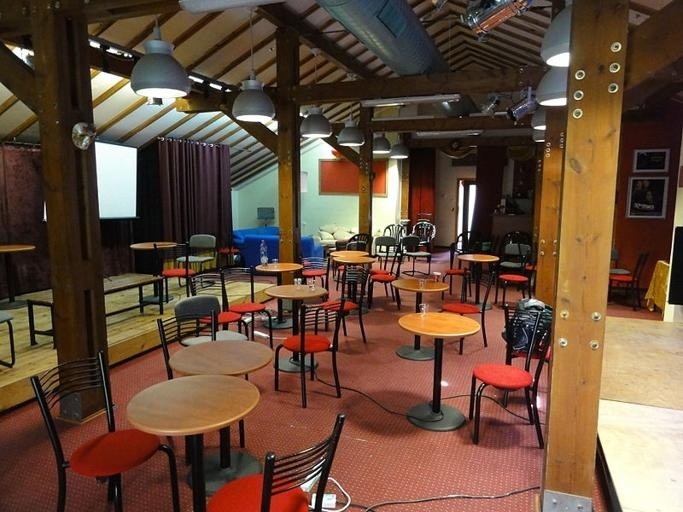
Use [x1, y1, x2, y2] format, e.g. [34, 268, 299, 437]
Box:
[464, 0, 533, 42]
[531, 105, 548, 131]
[256, 207, 275, 228]
[481, 93, 501, 118]
[130, 13, 192, 99]
[389, 132, 410, 160]
[539, 4, 571, 69]
[535, 66, 569, 108]
[506, 86, 537, 122]
[336, 73, 365, 147]
[371, 133, 392, 154]
[232, 7, 276, 123]
[299, 45, 333, 139]
[531, 128, 546, 143]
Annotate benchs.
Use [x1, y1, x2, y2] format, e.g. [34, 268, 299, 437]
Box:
[14, 272, 166, 346]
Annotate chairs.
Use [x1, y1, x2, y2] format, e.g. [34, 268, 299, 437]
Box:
[274, 298, 346, 408]
[348, 233, 369, 244]
[365, 251, 397, 300]
[367, 250, 401, 311]
[495, 241, 532, 292]
[406, 221, 436, 261]
[502, 303, 551, 408]
[30, 348, 180, 512]
[314, 266, 368, 343]
[438, 270, 496, 356]
[220, 265, 273, 349]
[378, 223, 407, 263]
[608, 251, 649, 311]
[0, 311, 16, 368]
[441, 242, 473, 300]
[611, 248, 630, 296]
[175, 233, 217, 288]
[495, 250, 537, 306]
[516, 250, 537, 293]
[301, 256, 330, 290]
[402, 236, 432, 277]
[374, 236, 402, 271]
[206, 413, 347, 512]
[173, 296, 248, 346]
[336, 241, 367, 292]
[189, 270, 249, 338]
[217, 245, 240, 269]
[468, 310, 552, 449]
[455, 231, 478, 269]
[156, 309, 216, 380]
[153, 241, 197, 303]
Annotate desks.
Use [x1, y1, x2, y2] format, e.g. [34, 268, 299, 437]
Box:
[643, 258, 670, 320]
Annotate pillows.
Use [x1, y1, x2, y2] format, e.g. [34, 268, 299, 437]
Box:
[319, 224, 337, 234]
[318, 231, 334, 240]
[333, 226, 351, 241]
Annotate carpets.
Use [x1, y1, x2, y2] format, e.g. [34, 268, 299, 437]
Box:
[600, 316, 683, 410]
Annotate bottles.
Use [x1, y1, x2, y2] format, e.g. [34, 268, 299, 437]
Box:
[259, 240, 269, 268]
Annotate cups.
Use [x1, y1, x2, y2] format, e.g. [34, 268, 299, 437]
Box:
[272, 259, 278, 267]
[417, 279, 427, 289]
[419, 303, 430, 317]
[308, 280, 316, 292]
[294, 278, 302, 290]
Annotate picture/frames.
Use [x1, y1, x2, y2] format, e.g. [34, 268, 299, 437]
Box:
[631, 147, 671, 173]
[624, 175, 670, 220]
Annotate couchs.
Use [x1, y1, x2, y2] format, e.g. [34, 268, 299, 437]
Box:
[312, 227, 357, 246]
[233, 227, 280, 239]
[232, 234, 324, 276]
